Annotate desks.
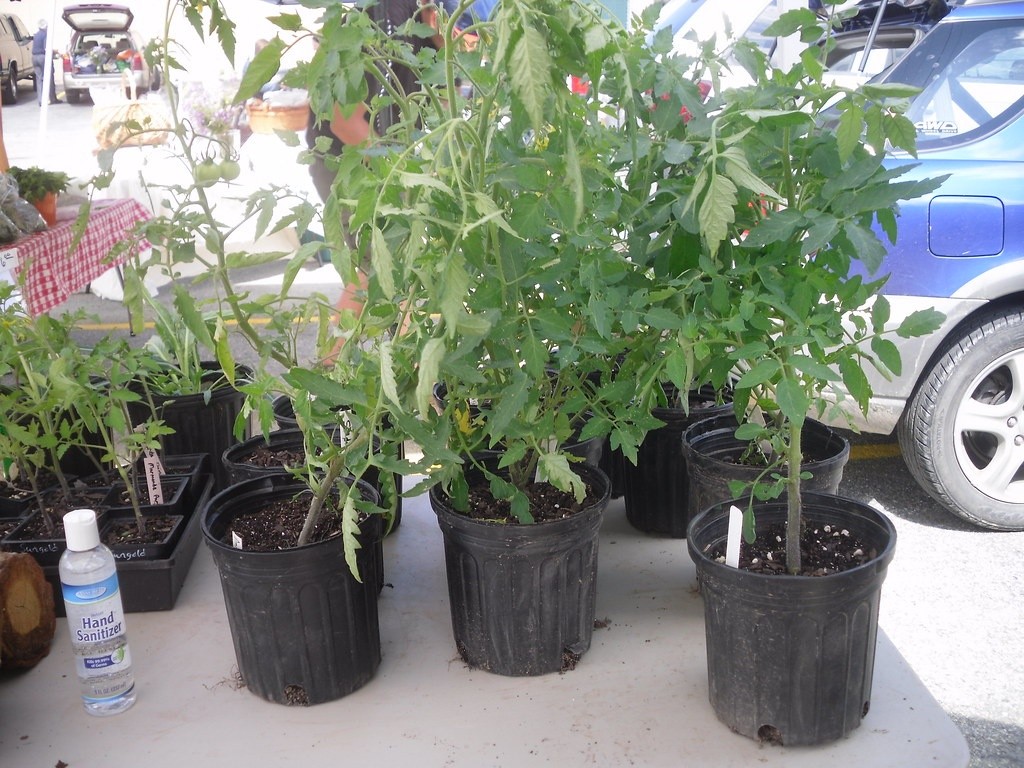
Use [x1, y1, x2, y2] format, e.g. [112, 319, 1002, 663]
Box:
[0, 454, 972, 768]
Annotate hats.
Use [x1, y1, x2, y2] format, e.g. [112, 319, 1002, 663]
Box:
[434, 0, 500, 35]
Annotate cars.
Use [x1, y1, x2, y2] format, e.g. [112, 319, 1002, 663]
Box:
[555, 1, 1024, 533]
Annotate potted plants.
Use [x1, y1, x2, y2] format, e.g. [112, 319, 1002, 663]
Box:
[0, 0, 954, 752]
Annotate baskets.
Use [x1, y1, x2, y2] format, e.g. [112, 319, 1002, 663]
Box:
[92, 68, 171, 147]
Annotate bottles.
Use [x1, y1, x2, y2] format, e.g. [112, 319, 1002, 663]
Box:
[59, 510, 137, 716]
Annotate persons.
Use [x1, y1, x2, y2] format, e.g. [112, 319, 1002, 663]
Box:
[32, 19, 63, 107]
[305, 0, 445, 275]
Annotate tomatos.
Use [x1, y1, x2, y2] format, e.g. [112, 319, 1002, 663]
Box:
[220, 160, 240, 179]
[197, 164, 220, 187]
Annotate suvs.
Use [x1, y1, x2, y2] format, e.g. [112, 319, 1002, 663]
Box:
[61, 3, 161, 104]
[0, 12, 37, 105]
